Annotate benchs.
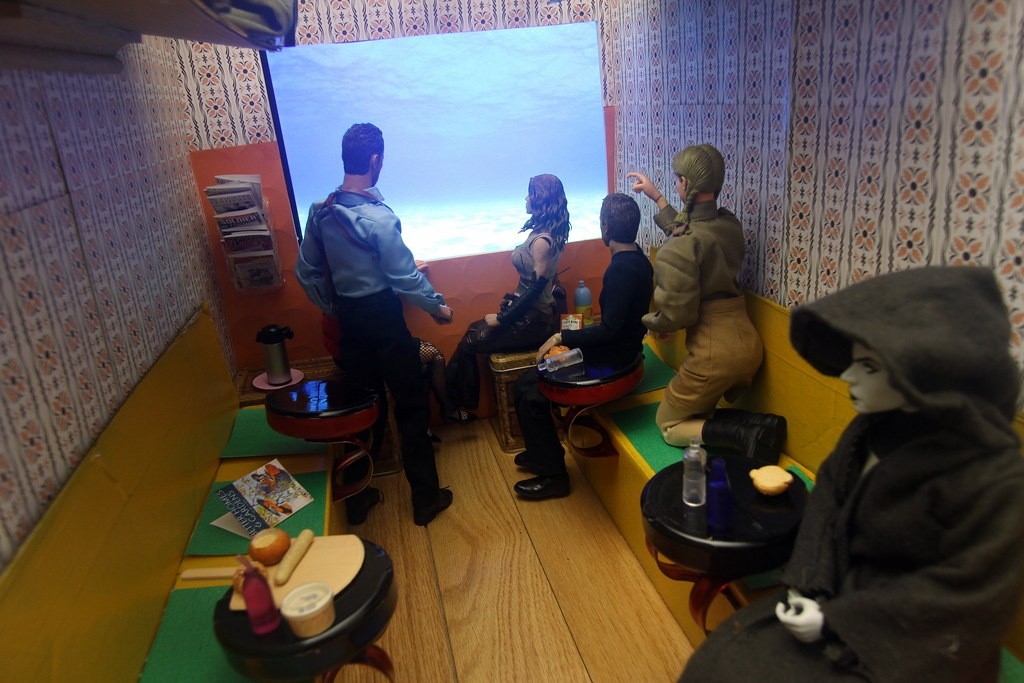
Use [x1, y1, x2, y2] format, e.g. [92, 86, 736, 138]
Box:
[563, 248, 859, 652]
[1, 303, 343, 683]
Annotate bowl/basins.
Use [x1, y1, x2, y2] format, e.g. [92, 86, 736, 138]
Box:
[281, 583, 335, 638]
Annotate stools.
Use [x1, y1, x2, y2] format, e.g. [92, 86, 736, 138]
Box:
[538, 362, 646, 457]
[266, 380, 379, 503]
[489, 346, 538, 453]
[640, 455, 809, 639]
[213, 536, 399, 682]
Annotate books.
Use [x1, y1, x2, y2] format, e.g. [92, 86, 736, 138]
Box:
[208, 458, 314, 539]
[205, 173, 282, 291]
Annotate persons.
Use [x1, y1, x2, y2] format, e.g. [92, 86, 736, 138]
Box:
[324, 142, 765, 499]
[296, 122, 453, 525]
[678, 267, 1024, 683]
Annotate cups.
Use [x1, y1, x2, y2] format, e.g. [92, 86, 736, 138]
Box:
[255, 324, 293, 386]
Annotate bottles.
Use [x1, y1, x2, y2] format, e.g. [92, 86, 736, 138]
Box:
[241, 569, 280, 635]
[683, 437, 707, 507]
[538, 348, 583, 373]
[574, 280, 595, 329]
[552, 280, 568, 330]
